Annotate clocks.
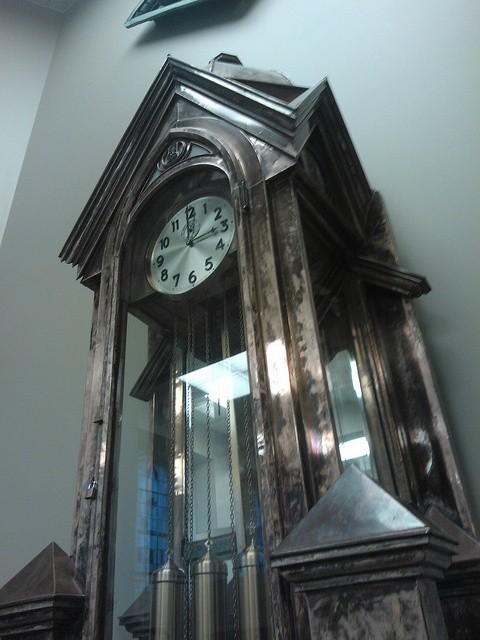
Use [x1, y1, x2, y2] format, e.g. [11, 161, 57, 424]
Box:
[0, 53, 480, 636]
